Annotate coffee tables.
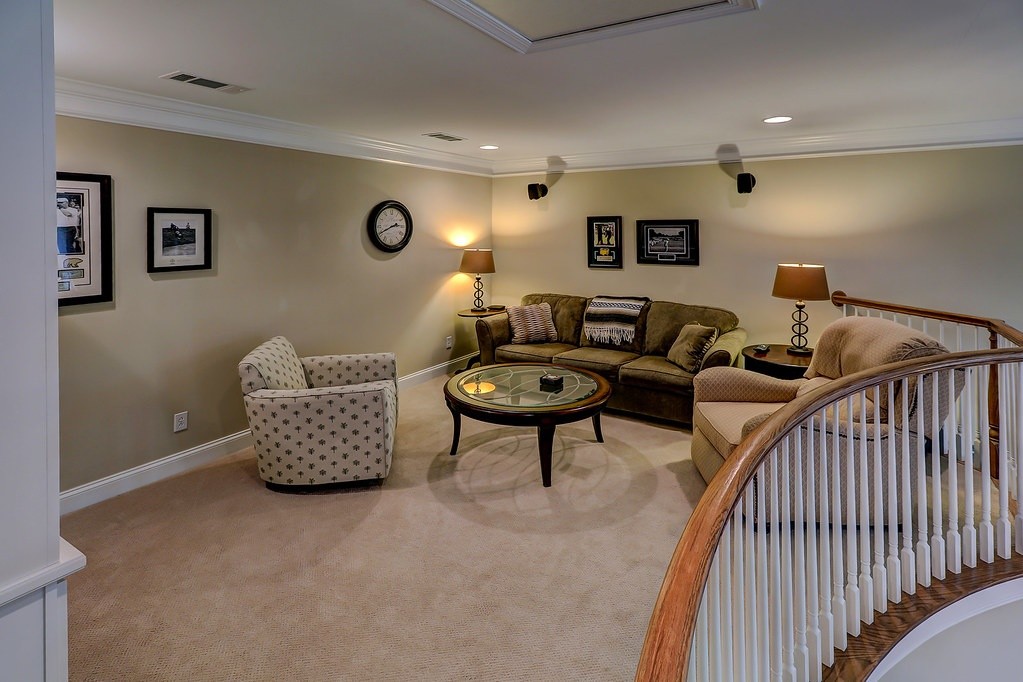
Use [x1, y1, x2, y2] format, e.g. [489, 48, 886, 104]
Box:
[438, 365, 610, 487]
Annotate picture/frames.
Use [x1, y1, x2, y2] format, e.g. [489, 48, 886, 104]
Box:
[587, 215, 623, 268]
[56, 173, 114, 306]
[635, 218, 700, 266]
[147, 206, 213, 274]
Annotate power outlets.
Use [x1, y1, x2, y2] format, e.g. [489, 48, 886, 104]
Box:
[173, 412, 189, 433]
[447, 336, 453, 349]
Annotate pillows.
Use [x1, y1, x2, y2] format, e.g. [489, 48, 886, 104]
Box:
[505, 302, 556, 342]
[668, 324, 718, 373]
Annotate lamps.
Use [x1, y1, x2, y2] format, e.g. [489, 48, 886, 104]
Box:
[771, 263, 831, 356]
[736, 172, 757, 196]
[458, 249, 494, 311]
[528, 182, 548, 201]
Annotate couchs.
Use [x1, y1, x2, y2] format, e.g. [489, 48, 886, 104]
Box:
[475, 292, 748, 429]
[689, 321, 958, 533]
[235, 335, 397, 491]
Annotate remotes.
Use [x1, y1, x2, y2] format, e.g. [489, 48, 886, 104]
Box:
[754, 344, 770, 352]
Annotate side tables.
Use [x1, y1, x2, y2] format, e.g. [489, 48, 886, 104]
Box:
[741, 343, 816, 380]
[454, 308, 505, 376]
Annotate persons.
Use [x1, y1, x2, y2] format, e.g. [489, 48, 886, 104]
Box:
[185, 222, 190, 229]
[57, 197, 83, 253]
[596, 225, 604, 244]
[664, 238, 670, 251]
[170, 222, 178, 233]
[603, 225, 612, 244]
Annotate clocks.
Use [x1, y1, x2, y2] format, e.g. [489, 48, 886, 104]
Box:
[365, 201, 413, 253]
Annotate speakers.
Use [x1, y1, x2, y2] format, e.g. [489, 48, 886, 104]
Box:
[528, 183, 541, 200]
[737, 173, 752, 194]
